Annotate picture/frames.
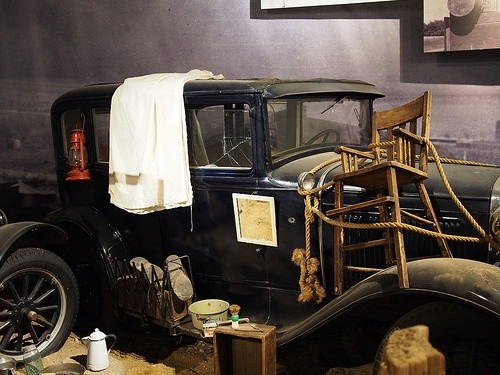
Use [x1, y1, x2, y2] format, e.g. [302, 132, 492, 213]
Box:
[412, 0, 500, 63]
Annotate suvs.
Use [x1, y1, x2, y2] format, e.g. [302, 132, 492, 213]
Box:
[0, 206, 79, 366]
[48, 78, 499, 375]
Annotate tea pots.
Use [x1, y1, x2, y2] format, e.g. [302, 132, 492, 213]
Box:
[82, 328, 117, 372]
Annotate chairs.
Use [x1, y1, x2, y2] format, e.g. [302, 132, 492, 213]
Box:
[325, 90, 453, 296]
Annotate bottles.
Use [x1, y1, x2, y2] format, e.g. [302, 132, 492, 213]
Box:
[23, 345, 44, 375]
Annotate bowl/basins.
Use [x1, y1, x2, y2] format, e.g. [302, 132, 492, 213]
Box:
[38, 363, 86, 375]
[188, 299, 230, 330]
